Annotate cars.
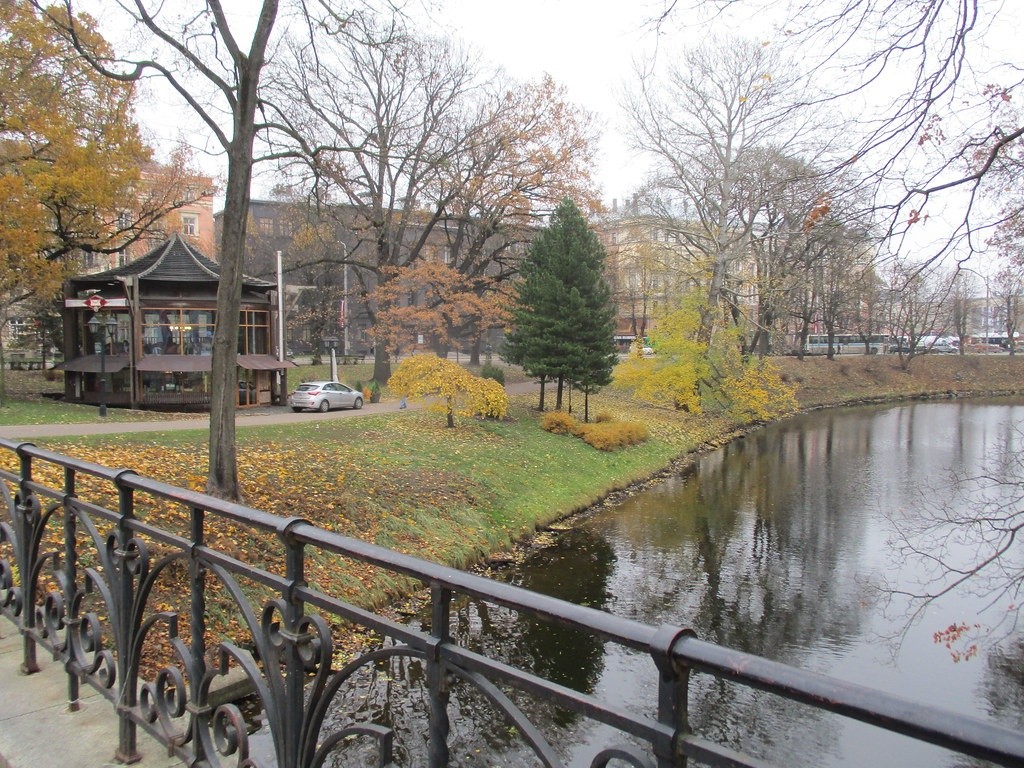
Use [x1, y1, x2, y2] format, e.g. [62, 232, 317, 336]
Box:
[889, 337, 917, 354]
[289, 380, 364, 413]
[927, 339, 959, 354]
[986, 345, 1005, 353]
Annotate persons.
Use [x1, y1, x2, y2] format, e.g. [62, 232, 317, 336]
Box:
[103, 319, 213, 392]
[400, 394, 407, 409]
[369, 346, 376, 360]
[836, 343, 841, 355]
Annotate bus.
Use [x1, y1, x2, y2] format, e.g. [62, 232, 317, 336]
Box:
[806, 332, 889, 356]
[968, 331, 1019, 353]
